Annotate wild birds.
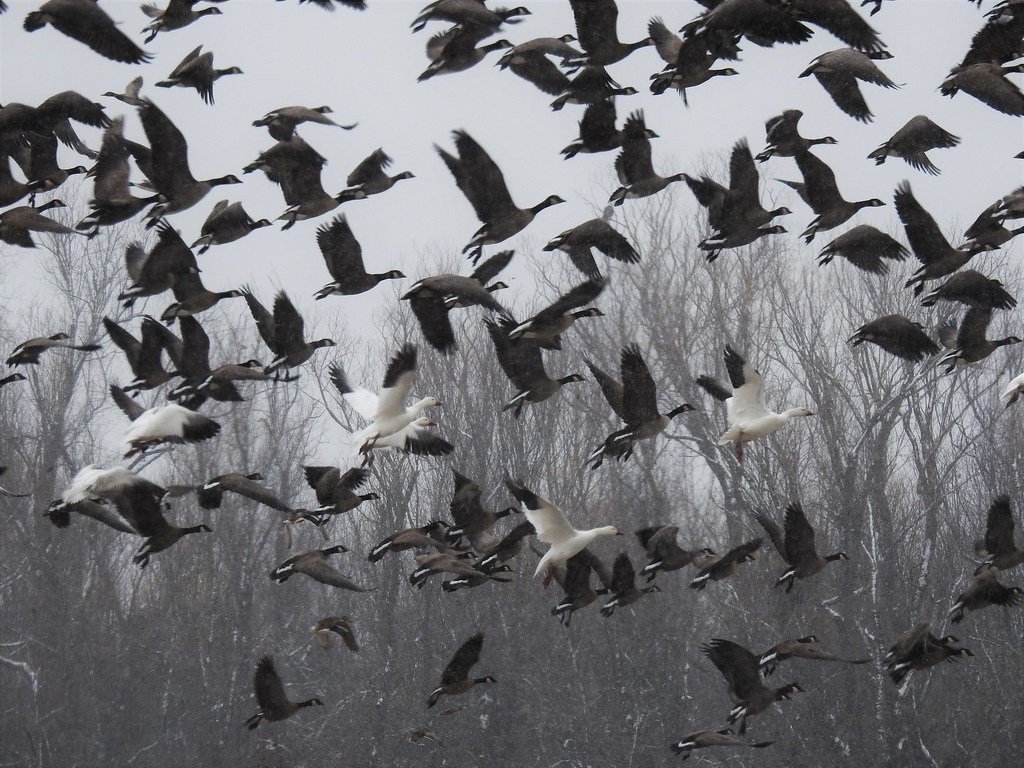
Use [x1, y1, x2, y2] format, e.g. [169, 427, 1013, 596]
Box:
[881, 622, 960, 672]
[590, 550, 663, 619]
[397, 720, 444, 746]
[309, 616, 360, 652]
[0, 0, 1024, 596]
[700, 638, 807, 734]
[946, 561, 1024, 625]
[525, 539, 615, 629]
[761, 635, 874, 680]
[244, 654, 325, 731]
[726, 685, 793, 725]
[670, 728, 776, 762]
[424, 631, 498, 709]
[882, 621, 975, 698]
